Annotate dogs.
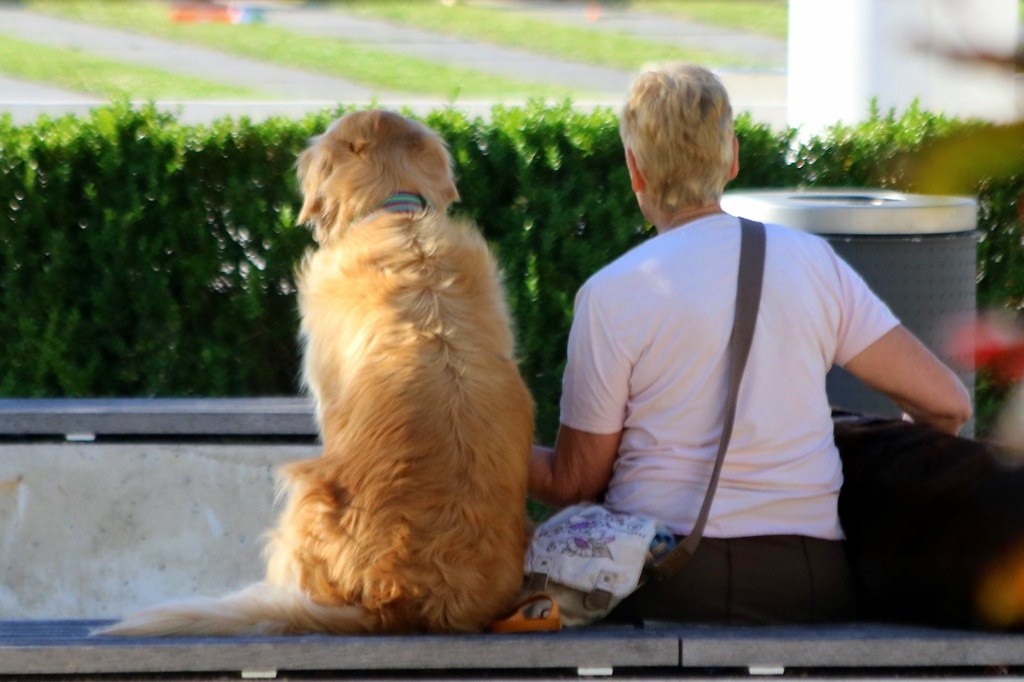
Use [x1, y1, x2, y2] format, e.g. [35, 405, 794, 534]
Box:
[87, 110, 538, 632]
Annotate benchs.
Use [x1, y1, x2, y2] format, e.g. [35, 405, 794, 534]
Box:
[0, 623, 1024, 682]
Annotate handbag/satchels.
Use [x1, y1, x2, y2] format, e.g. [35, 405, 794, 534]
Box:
[522, 501, 662, 629]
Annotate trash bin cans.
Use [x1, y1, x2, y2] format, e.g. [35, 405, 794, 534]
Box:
[720, 190, 978, 441]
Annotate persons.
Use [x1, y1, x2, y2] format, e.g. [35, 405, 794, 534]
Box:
[524, 63, 972, 629]
